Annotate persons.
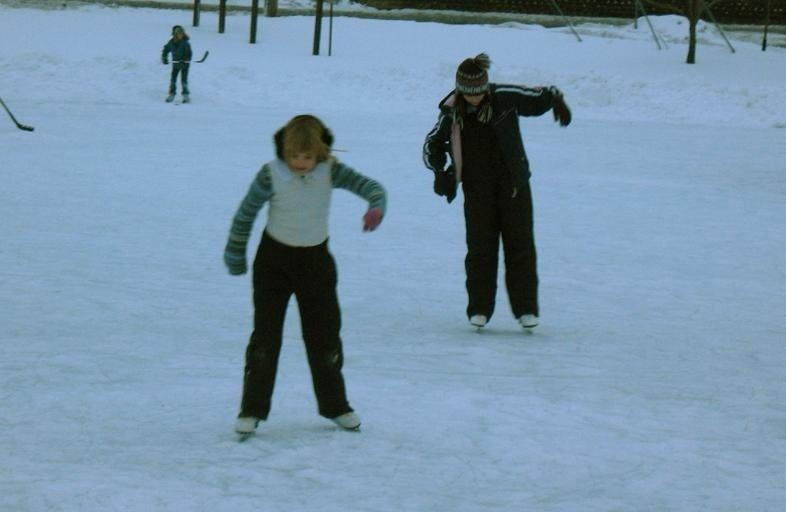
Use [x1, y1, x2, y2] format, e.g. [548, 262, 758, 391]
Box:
[219, 113, 388, 436]
[159, 24, 192, 102]
[419, 51, 573, 329]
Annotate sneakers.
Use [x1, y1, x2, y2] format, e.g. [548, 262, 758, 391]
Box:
[332, 412, 362, 430]
[182, 94, 190, 104]
[164, 94, 177, 103]
[520, 313, 538, 329]
[232, 417, 259, 435]
[469, 314, 487, 328]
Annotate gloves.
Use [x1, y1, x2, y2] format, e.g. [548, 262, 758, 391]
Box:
[550, 93, 573, 128]
[359, 210, 384, 233]
[433, 170, 449, 197]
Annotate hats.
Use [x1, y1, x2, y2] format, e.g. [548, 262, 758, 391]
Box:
[455, 59, 490, 96]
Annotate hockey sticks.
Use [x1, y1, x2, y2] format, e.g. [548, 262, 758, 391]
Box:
[165, 50, 210, 63]
[0, 98, 35, 132]
